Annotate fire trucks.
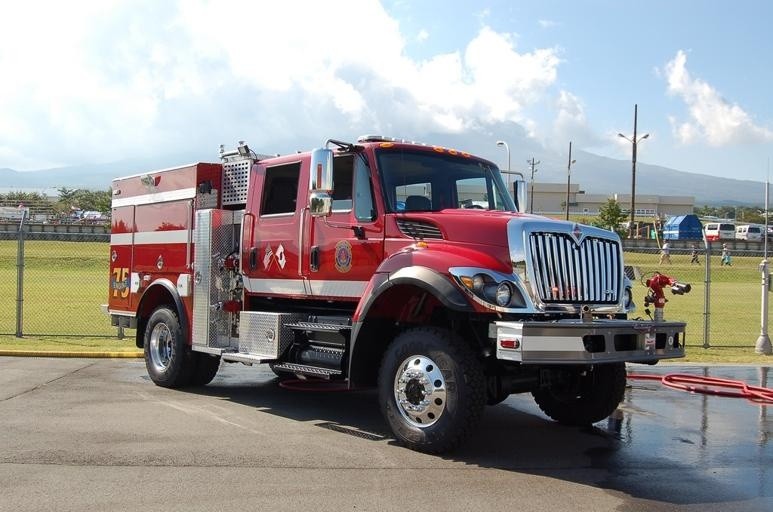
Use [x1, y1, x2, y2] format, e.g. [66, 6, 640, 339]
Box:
[108, 135, 692, 453]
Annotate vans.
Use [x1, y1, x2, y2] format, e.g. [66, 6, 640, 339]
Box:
[703, 222, 736, 241]
[735, 225, 761, 242]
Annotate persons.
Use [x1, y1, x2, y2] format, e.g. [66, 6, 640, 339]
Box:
[659, 239, 670, 265]
[690, 245, 703, 265]
[721, 244, 733, 266]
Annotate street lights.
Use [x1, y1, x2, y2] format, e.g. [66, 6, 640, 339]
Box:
[565, 141, 577, 221]
[616, 103, 648, 237]
[496, 140, 511, 190]
[526, 155, 541, 214]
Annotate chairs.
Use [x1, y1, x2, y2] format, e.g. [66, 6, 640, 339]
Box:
[402, 195, 432, 213]
